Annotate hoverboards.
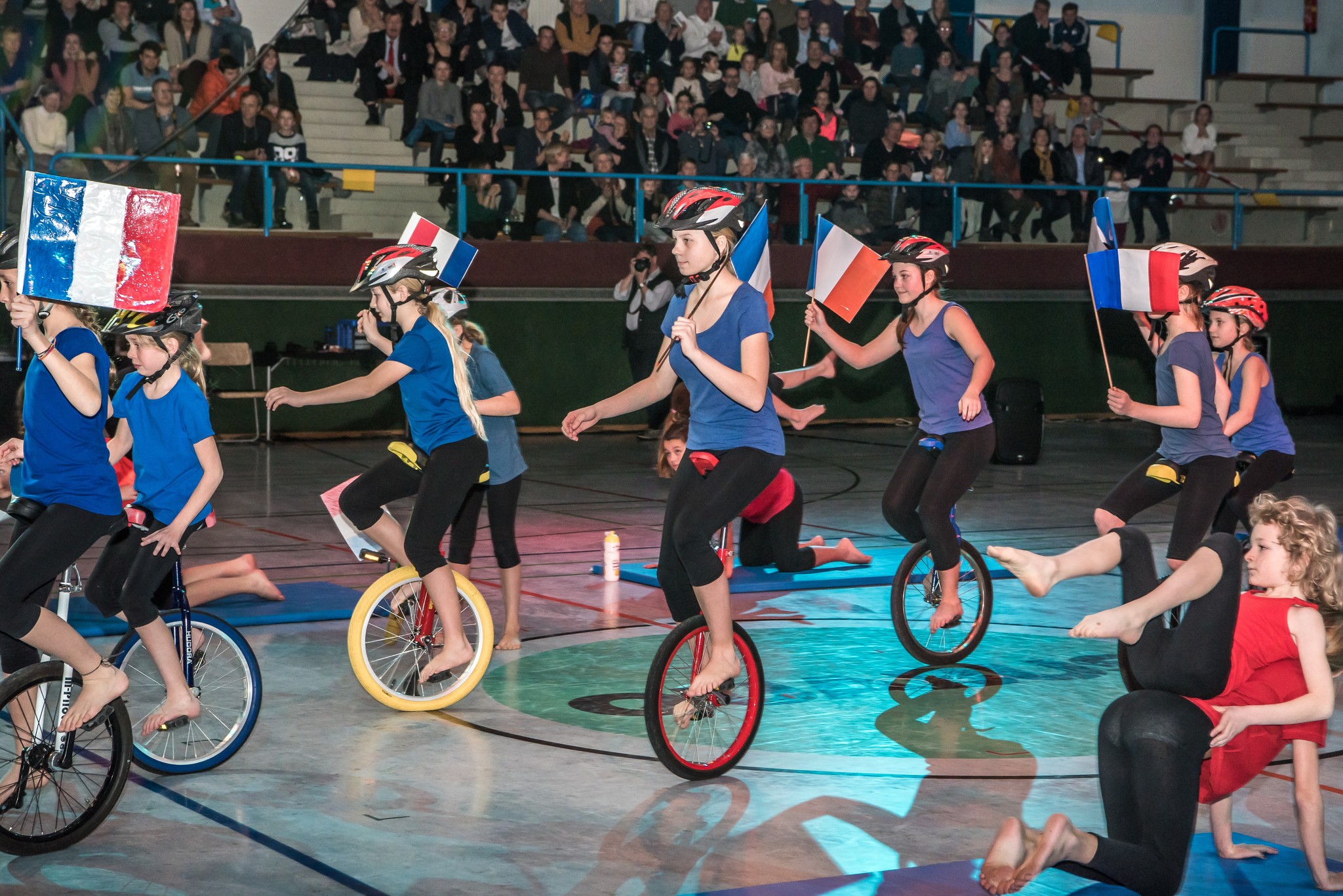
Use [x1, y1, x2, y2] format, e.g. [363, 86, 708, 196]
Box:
[345, 440, 497, 711]
[1, 487, 132, 852]
[1117, 459, 1248, 687]
[102, 479, 265, 776]
[642, 447, 767, 778]
[890, 431, 997, 666]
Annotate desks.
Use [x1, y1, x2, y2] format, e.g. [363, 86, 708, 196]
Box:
[249, 346, 418, 442]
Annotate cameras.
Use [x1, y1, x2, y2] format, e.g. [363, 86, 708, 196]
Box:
[634, 257, 651, 273]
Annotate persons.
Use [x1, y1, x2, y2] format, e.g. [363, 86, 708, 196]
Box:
[643, 419, 873, 582]
[1202, 284, 1297, 534]
[422, 286, 530, 651]
[0, 439, 286, 612]
[985, 492, 1343, 749]
[613, 243, 676, 440]
[0, 1, 1215, 250]
[979, 555, 1343, 896]
[84, 289, 223, 736]
[264, 245, 489, 683]
[804, 235, 995, 635]
[0, 221, 129, 805]
[657, 349, 838, 479]
[561, 186, 786, 728]
[1093, 242, 1238, 572]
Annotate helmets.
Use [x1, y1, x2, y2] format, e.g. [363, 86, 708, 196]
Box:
[0, 221, 21, 270]
[877, 235, 951, 277]
[348, 243, 439, 293]
[427, 287, 470, 323]
[100, 288, 203, 337]
[651, 187, 747, 232]
[1150, 242, 1218, 292]
[1197, 285, 1268, 331]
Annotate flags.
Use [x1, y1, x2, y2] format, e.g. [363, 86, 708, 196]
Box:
[396, 213, 479, 289]
[1087, 197, 1116, 253]
[730, 205, 775, 323]
[806, 217, 892, 323]
[17, 170, 181, 313]
[1082, 248, 1180, 312]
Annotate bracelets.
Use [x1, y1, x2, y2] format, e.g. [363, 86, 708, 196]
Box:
[637, 282, 645, 288]
[36, 334, 56, 360]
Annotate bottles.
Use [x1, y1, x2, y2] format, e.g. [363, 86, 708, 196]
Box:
[504, 217, 512, 236]
[325, 317, 337, 347]
[604, 530, 621, 581]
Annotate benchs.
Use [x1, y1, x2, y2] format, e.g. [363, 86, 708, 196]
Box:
[0, 1, 1342, 251]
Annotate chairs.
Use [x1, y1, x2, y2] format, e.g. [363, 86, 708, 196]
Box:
[199, 341, 269, 443]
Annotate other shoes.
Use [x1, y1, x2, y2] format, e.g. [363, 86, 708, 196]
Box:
[0, 0, 1219, 243]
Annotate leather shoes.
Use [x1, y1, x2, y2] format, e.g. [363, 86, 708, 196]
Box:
[637, 428, 664, 439]
